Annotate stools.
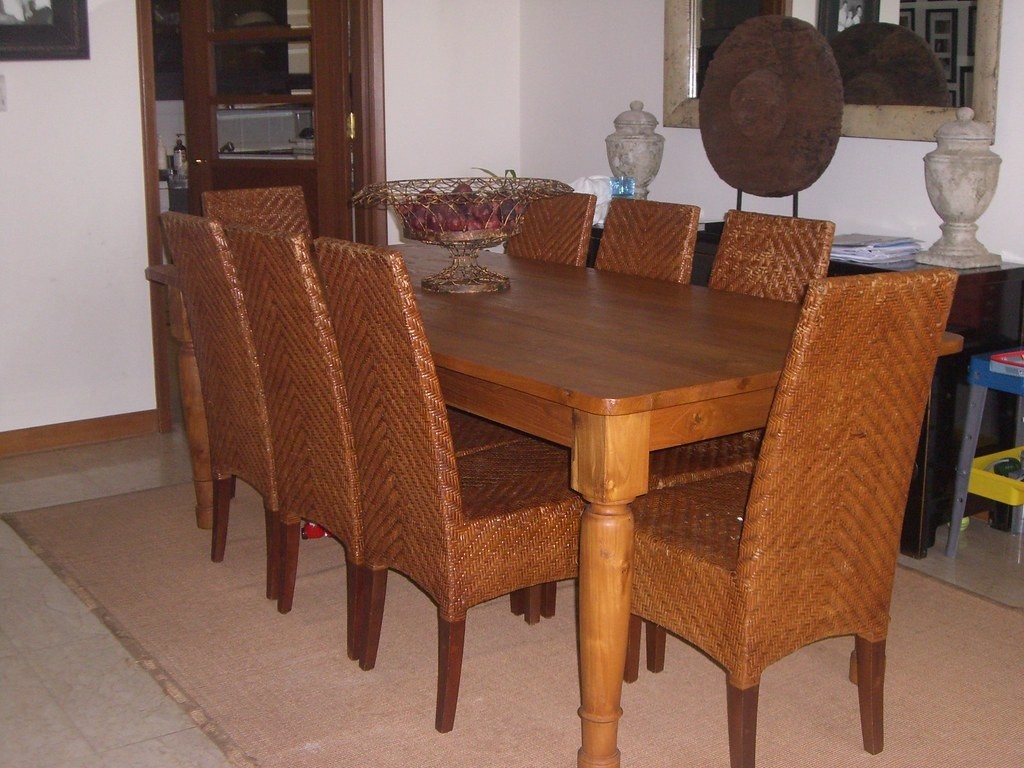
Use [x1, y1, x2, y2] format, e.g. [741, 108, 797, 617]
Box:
[945, 350, 1024, 557]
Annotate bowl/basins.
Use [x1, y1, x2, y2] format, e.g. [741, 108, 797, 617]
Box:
[233, 11, 275, 26]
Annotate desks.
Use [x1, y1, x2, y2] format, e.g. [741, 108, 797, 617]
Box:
[304, 244, 965, 768]
[588, 221, 1024, 559]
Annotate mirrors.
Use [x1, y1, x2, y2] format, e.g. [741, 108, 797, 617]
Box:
[662, 0, 1001, 145]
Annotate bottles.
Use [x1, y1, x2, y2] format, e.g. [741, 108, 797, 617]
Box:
[174, 140, 186, 174]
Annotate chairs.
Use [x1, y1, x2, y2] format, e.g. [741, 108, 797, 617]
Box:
[595, 200, 701, 285]
[161, 210, 285, 601]
[200, 183, 314, 244]
[621, 265, 962, 768]
[505, 194, 597, 267]
[641, 211, 835, 673]
[225, 221, 532, 661]
[315, 235, 592, 732]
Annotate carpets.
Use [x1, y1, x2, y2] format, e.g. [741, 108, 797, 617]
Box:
[0, 477, 1024, 768]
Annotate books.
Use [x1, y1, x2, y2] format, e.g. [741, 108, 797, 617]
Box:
[830, 232, 926, 264]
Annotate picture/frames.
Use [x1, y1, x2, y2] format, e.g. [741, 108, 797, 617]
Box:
[817, 0, 880, 43]
[0, 0, 91, 61]
